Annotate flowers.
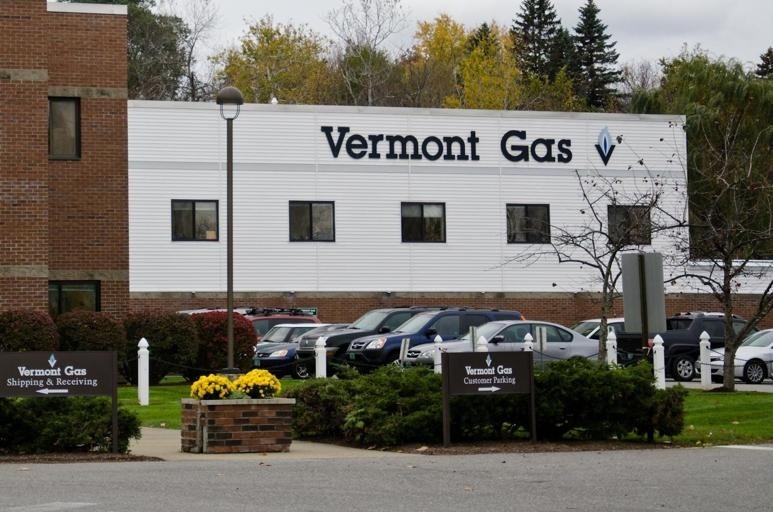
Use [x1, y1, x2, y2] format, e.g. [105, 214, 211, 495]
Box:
[232, 368, 282, 399]
[189, 374, 236, 399]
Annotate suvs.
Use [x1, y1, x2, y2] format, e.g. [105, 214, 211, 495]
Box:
[239, 307, 320, 344]
[297, 306, 431, 377]
[344, 308, 527, 371]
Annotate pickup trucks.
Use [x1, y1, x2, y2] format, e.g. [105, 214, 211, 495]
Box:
[616, 312, 761, 381]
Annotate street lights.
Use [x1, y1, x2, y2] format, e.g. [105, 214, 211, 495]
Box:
[214, 85, 243, 375]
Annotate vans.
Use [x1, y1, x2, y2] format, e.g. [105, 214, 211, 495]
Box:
[254, 323, 338, 352]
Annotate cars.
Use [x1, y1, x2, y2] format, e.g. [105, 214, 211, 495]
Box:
[571, 318, 626, 342]
[404, 318, 601, 369]
[252, 324, 349, 378]
[695, 329, 773, 386]
